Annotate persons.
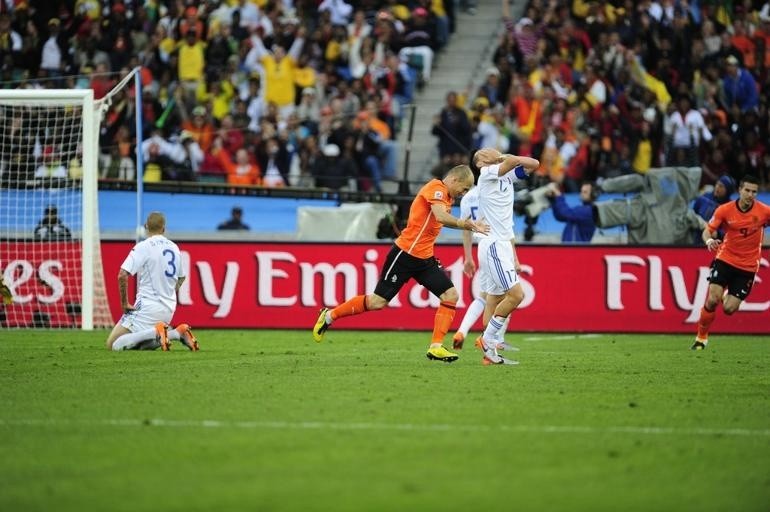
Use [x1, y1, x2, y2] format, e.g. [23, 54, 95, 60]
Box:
[310, 162, 491, 364]
[438, 1, 770, 248]
[103, 211, 203, 355]
[452, 179, 522, 353]
[35, 207, 71, 240]
[0, 0, 474, 197]
[217, 207, 249, 230]
[467, 144, 543, 366]
[685, 173, 770, 353]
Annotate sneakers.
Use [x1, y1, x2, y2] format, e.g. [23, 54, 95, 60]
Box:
[176, 323, 201, 352]
[313, 307, 334, 343]
[689, 334, 709, 351]
[426, 326, 522, 366]
[155, 321, 173, 352]
